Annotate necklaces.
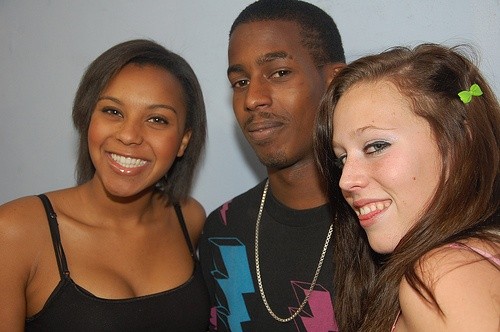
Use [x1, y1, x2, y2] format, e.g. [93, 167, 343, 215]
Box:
[254, 178, 338, 322]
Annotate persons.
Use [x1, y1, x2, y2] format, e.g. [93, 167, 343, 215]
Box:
[311, 37, 500, 332]
[0, 40, 216, 332]
[199, 0, 350, 332]
